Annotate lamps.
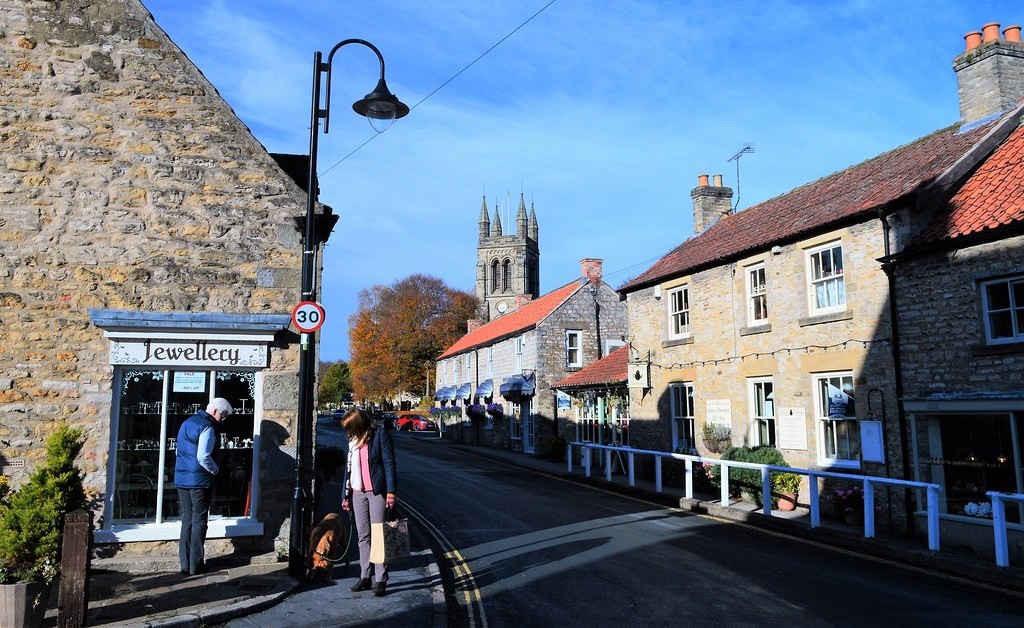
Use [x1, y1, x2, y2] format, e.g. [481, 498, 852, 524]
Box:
[771, 246, 782, 256]
[621, 336, 639, 356]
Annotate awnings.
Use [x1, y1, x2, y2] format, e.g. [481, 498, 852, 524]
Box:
[434, 373, 536, 400]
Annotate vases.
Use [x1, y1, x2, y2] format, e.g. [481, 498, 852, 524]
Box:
[494, 413, 499, 418]
[662, 473, 672, 487]
[843, 514, 860, 527]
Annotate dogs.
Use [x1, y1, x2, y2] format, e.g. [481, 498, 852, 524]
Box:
[305, 513, 350, 587]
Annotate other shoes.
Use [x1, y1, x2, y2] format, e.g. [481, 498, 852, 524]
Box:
[374, 581, 387, 596]
[351, 577, 372, 591]
[181, 567, 190, 575]
[189, 564, 210, 575]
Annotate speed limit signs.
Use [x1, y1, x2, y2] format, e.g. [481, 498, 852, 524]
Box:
[292, 301, 324, 332]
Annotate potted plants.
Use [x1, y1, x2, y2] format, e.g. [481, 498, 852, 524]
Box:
[0, 419, 94, 628]
[775, 473, 802, 512]
[570, 395, 626, 409]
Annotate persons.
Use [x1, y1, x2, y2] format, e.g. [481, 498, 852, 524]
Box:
[341, 410, 396, 595]
[174, 398, 232, 575]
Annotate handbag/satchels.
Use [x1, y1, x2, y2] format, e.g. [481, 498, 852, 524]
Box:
[382, 506, 411, 564]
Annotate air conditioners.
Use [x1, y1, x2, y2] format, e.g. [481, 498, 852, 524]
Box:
[606, 340, 629, 355]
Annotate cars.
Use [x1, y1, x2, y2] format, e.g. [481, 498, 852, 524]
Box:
[382, 410, 435, 431]
[333, 409, 346, 419]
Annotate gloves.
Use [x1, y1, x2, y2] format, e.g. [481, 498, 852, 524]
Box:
[342, 498, 350, 510]
[385, 492, 396, 509]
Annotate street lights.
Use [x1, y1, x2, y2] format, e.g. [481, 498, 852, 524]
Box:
[286, 39, 409, 581]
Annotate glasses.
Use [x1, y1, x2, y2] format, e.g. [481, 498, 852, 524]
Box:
[220, 413, 226, 421]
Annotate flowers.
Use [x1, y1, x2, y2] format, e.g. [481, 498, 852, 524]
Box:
[466, 403, 485, 421]
[964, 501, 992, 518]
[826, 486, 883, 527]
[486, 403, 503, 415]
[646, 460, 678, 475]
[694, 462, 715, 480]
[430, 405, 462, 419]
